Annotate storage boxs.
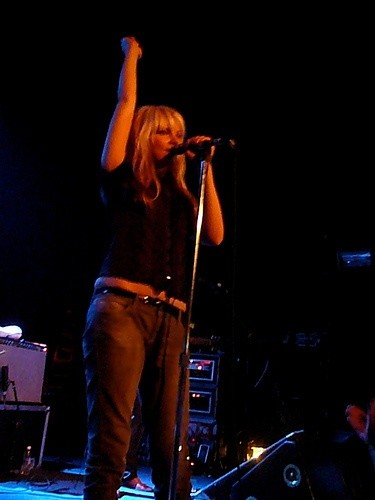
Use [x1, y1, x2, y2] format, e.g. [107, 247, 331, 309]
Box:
[1, 338, 50, 403]
[0, 403, 52, 477]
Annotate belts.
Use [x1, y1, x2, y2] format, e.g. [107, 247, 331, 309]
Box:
[94, 287, 187, 323]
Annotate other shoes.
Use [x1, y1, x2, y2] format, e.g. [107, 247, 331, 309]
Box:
[124, 477, 154, 495]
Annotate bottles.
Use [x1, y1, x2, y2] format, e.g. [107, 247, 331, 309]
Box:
[22, 445, 36, 477]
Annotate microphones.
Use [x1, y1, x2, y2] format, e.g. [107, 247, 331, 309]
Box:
[170, 138, 236, 156]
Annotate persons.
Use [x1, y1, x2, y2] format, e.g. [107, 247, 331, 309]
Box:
[78, 36, 223, 500]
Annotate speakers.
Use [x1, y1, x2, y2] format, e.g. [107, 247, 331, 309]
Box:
[203, 429, 374, 499]
